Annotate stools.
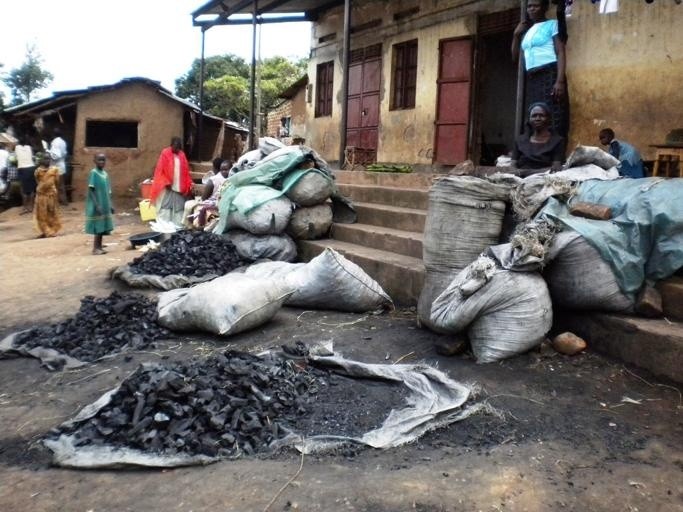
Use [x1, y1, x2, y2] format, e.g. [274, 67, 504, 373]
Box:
[341, 146, 377, 171]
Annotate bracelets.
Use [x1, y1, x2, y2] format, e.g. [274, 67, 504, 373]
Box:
[557, 80, 566, 83]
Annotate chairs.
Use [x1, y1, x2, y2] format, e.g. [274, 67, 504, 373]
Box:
[648, 129, 683, 177]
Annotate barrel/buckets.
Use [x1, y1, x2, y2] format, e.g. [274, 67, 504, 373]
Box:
[138, 199, 156, 221]
[140, 182, 152, 199]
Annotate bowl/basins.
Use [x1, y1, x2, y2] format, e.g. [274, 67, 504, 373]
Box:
[127, 231, 161, 248]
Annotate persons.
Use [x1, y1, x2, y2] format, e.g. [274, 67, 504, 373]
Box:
[511, 102, 565, 173]
[0, 123, 233, 254]
[599, 128, 644, 178]
[511, 2, 569, 163]
